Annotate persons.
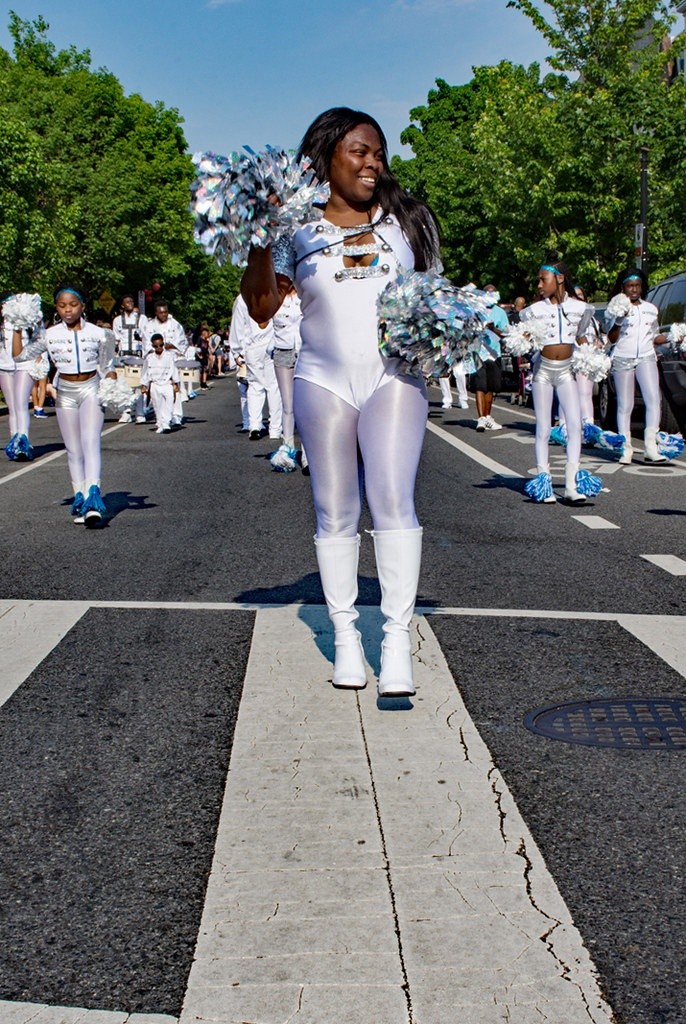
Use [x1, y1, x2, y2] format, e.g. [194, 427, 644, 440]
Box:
[439, 360, 468, 409]
[228, 295, 282, 438]
[608, 268, 682, 463]
[559, 286, 607, 448]
[509, 297, 526, 404]
[0, 318, 59, 459]
[259, 285, 309, 470]
[102, 294, 223, 432]
[241, 108, 443, 695]
[471, 285, 510, 431]
[12, 283, 118, 523]
[519, 261, 600, 503]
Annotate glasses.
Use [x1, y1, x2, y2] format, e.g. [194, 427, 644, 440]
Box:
[154, 343, 163, 347]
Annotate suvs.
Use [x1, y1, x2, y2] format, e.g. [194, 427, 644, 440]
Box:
[453, 303, 520, 390]
[594, 269, 686, 440]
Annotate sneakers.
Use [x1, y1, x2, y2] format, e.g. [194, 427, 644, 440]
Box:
[485, 415, 502, 430]
[475, 417, 488, 431]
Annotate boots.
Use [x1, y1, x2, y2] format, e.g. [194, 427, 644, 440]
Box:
[70, 481, 86, 524]
[83, 480, 106, 526]
[301, 443, 310, 476]
[6, 432, 33, 461]
[281, 436, 295, 453]
[643, 428, 666, 462]
[33, 409, 48, 418]
[364, 526, 423, 697]
[617, 431, 634, 464]
[525, 463, 557, 503]
[564, 463, 602, 503]
[313, 533, 367, 690]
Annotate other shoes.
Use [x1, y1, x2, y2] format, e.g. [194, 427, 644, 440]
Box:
[442, 404, 452, 409]
[218, 372, 225, 375]
[171, 422, 181, 428]
[242, 425, 250, 431]
[458, 401, 468, 409]
[136, 417, 146, 423]
[252, 429, 266, 441]
[201, 384, 209, 390]
[163, 427, 171, 432]
[119, 414, 132, 422]
[156, 427, 163, 433]
